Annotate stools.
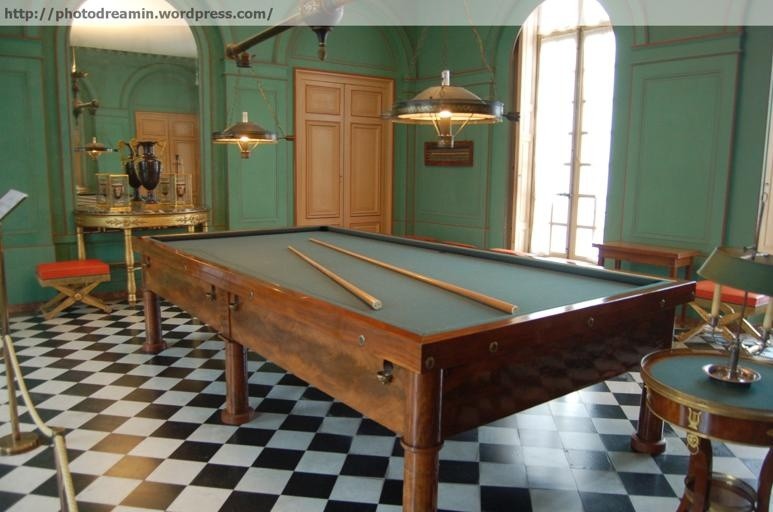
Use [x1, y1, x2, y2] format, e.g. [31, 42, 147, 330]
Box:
[675, 279, 772, 359]
[35, 258, 113, 321]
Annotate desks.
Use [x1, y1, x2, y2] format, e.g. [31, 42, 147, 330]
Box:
[133, 224, 698, 511]
[592, 241, 704, 328]
[74, 211, 208, 306]
[637, 347, 773, 511]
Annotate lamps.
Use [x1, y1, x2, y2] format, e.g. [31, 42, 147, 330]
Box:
[697, 191, 772, 387]
[68, 47, 113, 166]
[209, 0, 506, 160]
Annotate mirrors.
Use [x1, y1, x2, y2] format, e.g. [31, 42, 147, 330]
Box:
[67, 0, 205, 215]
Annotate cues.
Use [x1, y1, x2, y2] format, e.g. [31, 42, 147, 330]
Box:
[289, 246, 381, 311]
[309, 239, 517, 315]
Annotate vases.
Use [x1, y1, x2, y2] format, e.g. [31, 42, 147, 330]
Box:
[134, 140, 161, 205]
[115, 142, 145, 203]
